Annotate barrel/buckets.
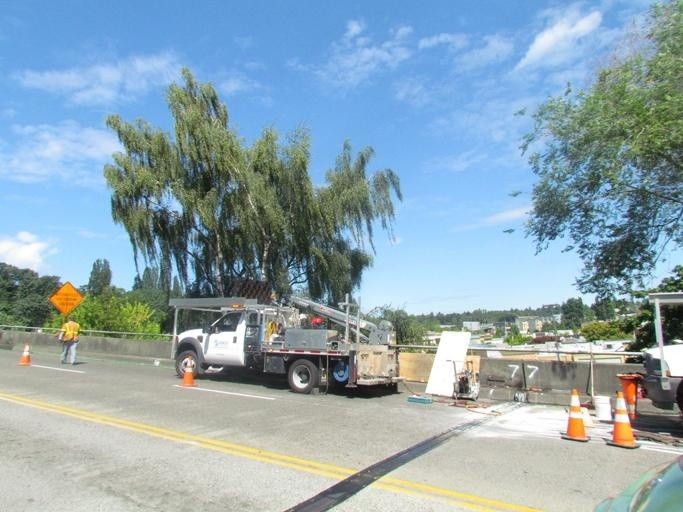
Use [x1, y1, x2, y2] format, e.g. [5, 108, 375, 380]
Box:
[592, 393, 615, 424]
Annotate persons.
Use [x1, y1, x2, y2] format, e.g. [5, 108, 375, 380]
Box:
[57, 314, 80, 366]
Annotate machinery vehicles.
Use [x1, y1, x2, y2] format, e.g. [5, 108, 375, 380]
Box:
[167, 292, 402, 395]
[637, 290, 683, 416]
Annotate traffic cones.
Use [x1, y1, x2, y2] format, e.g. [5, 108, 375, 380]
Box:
[175, 353, 196, 387]
[615, 372, 645, 421]
[17, 342, 33, 368]
[603, 389, 641, 449]
[560, 388, 592, 442]
